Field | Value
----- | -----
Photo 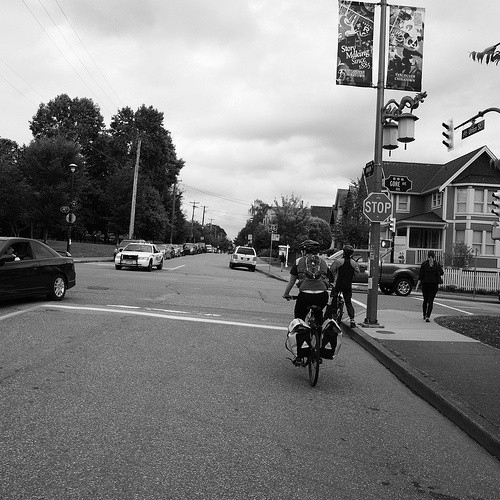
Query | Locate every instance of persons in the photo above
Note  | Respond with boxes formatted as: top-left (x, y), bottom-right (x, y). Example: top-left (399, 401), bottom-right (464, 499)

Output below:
top-left (5, 246), bottom-right (27, 260)
top-left (323, 245), bottom-right (360, 328)
top-left (416, 250), bottom-right (445, 323)
top-left (280, 252), bottom-right (285, 271)
top-left (183, 244), bottom-right (186, 250)
top-left (398, 252), bottom-right (404, 263)
top-left (284, 240), bottom-right (335, 366)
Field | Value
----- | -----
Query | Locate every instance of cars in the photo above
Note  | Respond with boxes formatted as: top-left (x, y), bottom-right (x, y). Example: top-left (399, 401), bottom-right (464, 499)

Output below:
top-left (158, 243), bottom-right (207, 260)
top-left (115, 242), bottom-right (164, 271)
top-left (0, 236), bottom-right (77, 301)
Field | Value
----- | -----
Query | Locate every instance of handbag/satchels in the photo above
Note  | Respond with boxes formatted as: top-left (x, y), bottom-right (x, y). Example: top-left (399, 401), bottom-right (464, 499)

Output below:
top-left (285, 318), bottom-right (317, 358)
top-left (437, 261), bottom-right (443, 284)
top-left (319, 318), bottom-right (343, 360)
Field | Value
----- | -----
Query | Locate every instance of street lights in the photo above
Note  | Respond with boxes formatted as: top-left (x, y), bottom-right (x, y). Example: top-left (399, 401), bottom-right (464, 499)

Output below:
top-left (67, 164), bottom-right (78, 253)
top-left (358, 90), bottom-right (428, 330)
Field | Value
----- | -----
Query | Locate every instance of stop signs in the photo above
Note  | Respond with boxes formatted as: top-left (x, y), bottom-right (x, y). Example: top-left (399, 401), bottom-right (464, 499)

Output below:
top-left (362, 192), bottom-right (393, 223)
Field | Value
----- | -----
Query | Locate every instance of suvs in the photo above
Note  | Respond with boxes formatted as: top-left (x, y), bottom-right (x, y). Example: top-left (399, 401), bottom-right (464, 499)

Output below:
top-left (229, 246), bottom-right (257, 272)
top-left (324, 249), bottom-right (421, 297)
top-left (206, 245), bottom-right (212, 252)
top-left (113, 239), bottom-right (145, 261)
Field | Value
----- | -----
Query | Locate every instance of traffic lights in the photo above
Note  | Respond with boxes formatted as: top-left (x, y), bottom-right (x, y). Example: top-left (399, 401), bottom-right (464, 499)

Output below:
top-left (490, 191), bottom-right (500, 216)
top-left (442, 120), bottom-right (455, 151)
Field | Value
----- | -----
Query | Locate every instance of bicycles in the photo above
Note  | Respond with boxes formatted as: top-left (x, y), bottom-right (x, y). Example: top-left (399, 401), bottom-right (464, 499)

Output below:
top-left (323, 282), bottom-right (345, 330)
top-left (283, 294), bottom-right (323, 388)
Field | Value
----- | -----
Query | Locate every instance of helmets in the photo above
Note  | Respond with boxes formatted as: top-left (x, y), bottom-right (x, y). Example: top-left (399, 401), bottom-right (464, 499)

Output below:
top-left (343, 245), bottom-right (354, 253)
top-left (299, 240), bottom-right (320, 254)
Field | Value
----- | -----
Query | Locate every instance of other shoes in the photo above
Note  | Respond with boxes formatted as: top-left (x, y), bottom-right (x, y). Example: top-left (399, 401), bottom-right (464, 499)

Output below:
top-left (315, 357), bottom-right (322, 364)
top-left (295, 358), bottom-right (302, 364)
top-left (350, 320), bottom-right (356, 329)
top-left (425, 319), bottom-right (430, 322)
top-left (423, 316), bottom-right (426, 320)
